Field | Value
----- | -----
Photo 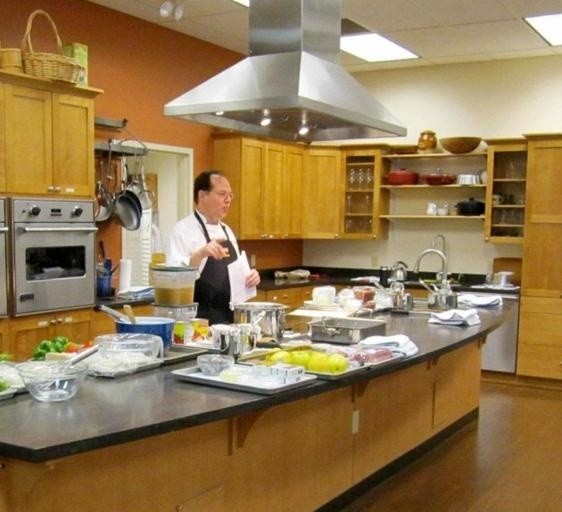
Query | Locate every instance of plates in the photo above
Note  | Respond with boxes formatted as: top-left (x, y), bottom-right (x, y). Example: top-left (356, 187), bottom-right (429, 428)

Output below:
top-left (171, 363), bottom-right (319, 396)
top-left (237, 346), bottom-right (373, 382)
top-left (54, 344), bottom-right (210, 379)
top-left (303, 301), bottom-right (341, 313)
top-left (0, 358), bottom-right (31, 403)
top-left (367, 350), bottom-right (406, 369)
top-left (175, 335), bottom-right (230, 354)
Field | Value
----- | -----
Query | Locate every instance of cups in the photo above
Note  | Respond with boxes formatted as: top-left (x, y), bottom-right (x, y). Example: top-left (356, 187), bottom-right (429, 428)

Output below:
top-left (492, 194), bottom-right (505, 207)
top-left (313, 286), bottom-right (337, 305)
top-left (347, 168), bottom-right (374, 188)
top-left (380, 267), bottom-right (389, 288)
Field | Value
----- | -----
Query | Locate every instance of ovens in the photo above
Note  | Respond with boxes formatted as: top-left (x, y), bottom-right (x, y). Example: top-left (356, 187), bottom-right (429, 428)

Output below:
top-left (0, 197), bottom-right (10, 318)
top-left (12, 197), bottom-right (96, 317)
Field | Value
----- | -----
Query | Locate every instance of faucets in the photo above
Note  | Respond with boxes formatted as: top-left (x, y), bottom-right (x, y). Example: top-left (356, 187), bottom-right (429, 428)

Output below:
top-left (432, 234), bottom-right (446, 256)
top-left (414, 250), bottom-right (448, 309)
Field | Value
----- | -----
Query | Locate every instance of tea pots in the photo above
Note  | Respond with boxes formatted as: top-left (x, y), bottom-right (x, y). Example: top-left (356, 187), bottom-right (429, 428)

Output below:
top-left (427, 202), bottom-right (438, 217)
top-left (372, 280), bottom-right (414, 313)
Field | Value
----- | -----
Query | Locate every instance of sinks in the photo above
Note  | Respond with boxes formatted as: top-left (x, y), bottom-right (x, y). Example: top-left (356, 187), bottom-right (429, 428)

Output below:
top-left (411, 301), bottom-right (437, 312)
top-left (405, 280), bottom-right (460, 286)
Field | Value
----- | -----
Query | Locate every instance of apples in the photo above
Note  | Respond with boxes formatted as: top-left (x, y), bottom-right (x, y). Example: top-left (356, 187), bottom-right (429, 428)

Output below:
top-left (326, 354), bottom-right (348, 374)
top-left (288, 350), bottom-right (310, 366)
top-left (307, 351), bottom-right (330, 373)
top-left (269, 349), bottom-right (288, 365)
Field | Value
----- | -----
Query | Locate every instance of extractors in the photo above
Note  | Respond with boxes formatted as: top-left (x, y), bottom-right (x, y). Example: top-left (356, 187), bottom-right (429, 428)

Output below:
top-left (163, 3), bottom-right (409, 145)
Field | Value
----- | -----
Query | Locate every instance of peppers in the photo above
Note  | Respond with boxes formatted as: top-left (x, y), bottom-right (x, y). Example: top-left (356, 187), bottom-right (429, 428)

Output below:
top-left (33, 335), bottom-right (69, 360)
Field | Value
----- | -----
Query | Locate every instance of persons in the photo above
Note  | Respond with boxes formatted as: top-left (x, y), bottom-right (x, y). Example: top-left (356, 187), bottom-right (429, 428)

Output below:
top-left (168, 172), bottom-right (260, 325)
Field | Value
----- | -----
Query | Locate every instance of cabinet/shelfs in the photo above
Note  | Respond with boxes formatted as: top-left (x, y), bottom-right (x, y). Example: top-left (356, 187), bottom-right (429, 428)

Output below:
top-left (334, 285), bottom-right (347, 296)
top-left (304, 149), bottom-right (388, 240)
top-left (0, 80), bottom-right (95, 198)
top-left (212, 137), bottom-right (306, 239)
top-left (9, 308), bottom-right (93, 364)
top-left (93, 305), bottom-right (154, 337)
top-left (403, 289), bottom-right (438, 301)
top-left (248, 287), bottom-right (325, 335)
top-left (379, 152), bottom-right (488, 220)
top-left (482, 138), bottom-right (528, 245)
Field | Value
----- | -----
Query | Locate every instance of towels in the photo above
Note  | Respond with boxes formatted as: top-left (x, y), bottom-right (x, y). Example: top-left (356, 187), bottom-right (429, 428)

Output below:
top-left (358, 334), bottom-right (419, 359)
top-left (457, 292), bottom-right (502, 308)
top-left (427, 308), bottom-right (481, 326)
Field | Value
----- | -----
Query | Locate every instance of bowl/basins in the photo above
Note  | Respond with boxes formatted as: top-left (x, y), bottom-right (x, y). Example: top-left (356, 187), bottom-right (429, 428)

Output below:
top-left (17, 359), bottom-right (90, 403)
top-left (438, 208), bottom-right (448, 215)
top-left (94, 333), bottom-right (162, 365)
top-left (196, 353), bottom-right (234, 376)
top-left (233, 302), bottom-right (286, 348)
top-left (454, 175), bottom-right (483, 189)
top-left (396, 146), bottom-right (417, 155)
top-left (311, 308), bottom-right (387, 346)
top-left (440, 137), bottom-right (482, 155)
top-left (115, 315), bottom-right (176, 357)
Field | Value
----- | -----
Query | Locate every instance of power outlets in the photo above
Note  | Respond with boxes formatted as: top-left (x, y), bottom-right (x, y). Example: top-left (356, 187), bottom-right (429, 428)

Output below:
top-left (250, 254), bottom-right (256, 267)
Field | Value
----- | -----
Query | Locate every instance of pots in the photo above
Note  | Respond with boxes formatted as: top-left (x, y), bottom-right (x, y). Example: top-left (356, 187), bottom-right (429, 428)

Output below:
top-left (116, 162), bottom-right (143, 234)
top-left (418, 131), bottom-right (438, 157)
top-left (418, 169), bottom-right (456, 187)
top-left (383, 167), bottom-right (419, 185)
top-left (95, 184), bottom-right (115, 223)
top-left (454, 198), bottom-right (486, 217)
